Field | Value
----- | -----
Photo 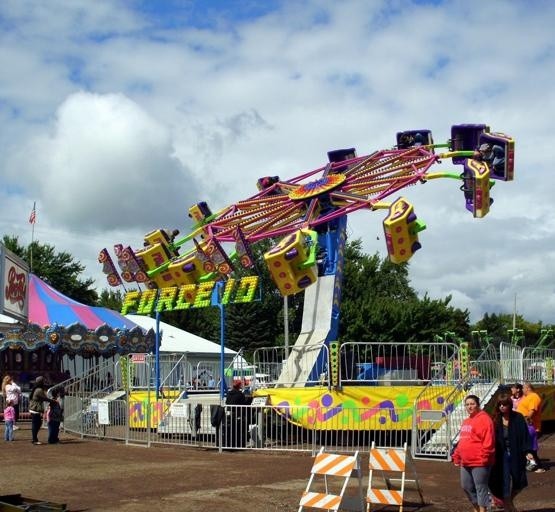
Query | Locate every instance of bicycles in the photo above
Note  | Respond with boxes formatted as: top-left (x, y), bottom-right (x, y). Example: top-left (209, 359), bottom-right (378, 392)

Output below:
top-left (77, 400), bottom-right (106, 441)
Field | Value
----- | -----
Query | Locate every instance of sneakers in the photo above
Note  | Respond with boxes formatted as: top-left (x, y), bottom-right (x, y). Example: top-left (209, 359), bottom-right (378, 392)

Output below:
top-left (32, 441), bottom-right (42, 445)
top-left (526, 464), bottom-right (545, 473)
top-left (13, 425), bottom-right (20, 430)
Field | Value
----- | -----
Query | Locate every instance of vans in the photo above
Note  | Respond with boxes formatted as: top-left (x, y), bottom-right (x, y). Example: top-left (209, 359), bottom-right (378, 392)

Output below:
top-left (431, 362), bottom-right (445, 371)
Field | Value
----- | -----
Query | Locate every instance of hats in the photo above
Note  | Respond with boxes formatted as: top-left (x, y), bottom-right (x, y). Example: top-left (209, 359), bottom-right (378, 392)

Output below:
top-left (36, 377), bottom-right (47, 384)
top-left (233, 380), bottom-right (241, 387)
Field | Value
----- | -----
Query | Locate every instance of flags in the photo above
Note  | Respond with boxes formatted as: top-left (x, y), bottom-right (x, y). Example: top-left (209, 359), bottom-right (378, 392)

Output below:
top-left (29, 203), bottom-right (36, 225)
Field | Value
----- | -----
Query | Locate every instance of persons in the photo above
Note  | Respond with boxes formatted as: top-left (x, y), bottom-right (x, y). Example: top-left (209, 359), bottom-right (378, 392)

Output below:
top-left (450, 395), bottom-right (497, 512)
top-left (508, 383), bottom-right (523, 411)
top-left (486, 392), bottom-right (536, 511)
top-left (515, 381), bottom-right (546, 474)
top-left (1, 368), bottom-right (247, 450)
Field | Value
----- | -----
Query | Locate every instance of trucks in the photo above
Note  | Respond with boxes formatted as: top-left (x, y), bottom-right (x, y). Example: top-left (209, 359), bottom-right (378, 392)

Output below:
top-left (525, 359), bottom-right (555, 381)
